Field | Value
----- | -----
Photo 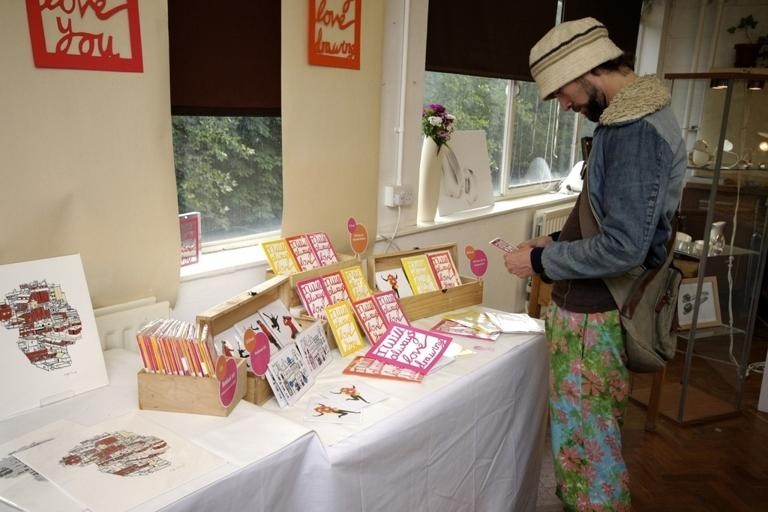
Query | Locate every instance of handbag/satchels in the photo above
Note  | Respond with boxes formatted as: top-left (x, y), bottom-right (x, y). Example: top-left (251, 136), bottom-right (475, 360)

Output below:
top-left (622, 264), bottom-right (684, 377)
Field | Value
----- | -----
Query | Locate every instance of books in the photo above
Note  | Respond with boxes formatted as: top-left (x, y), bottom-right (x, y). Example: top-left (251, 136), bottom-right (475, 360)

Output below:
top-left (136, 231), bottom-right (546, 409)
top-left (179, 212), bottom-right (201, 267)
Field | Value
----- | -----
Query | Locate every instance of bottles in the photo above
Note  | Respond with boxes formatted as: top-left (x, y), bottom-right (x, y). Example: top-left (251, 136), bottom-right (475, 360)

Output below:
top-left (706, 221), bottom-right (726, 257)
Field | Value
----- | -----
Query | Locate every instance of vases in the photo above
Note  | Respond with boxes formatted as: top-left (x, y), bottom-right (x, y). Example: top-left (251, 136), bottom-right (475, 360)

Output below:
top-left (417, 134), bottom-right (443, 223)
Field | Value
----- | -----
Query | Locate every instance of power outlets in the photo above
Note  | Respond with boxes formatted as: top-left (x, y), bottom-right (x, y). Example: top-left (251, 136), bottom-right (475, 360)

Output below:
top-left (384, 185), bottom-right (414, 208)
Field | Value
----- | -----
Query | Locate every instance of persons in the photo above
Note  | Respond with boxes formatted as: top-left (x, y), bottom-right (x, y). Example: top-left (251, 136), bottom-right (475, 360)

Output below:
top-left (502, 16), bottom-right (689, 510)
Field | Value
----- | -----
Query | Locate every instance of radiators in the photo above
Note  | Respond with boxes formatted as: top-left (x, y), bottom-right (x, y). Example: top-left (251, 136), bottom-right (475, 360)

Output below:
top-left (526, 201), bottom-right (578, 294)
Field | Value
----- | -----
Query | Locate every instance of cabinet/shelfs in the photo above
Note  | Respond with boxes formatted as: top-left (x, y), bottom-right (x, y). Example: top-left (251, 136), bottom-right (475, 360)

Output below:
top-left (621, 66), bottom-right (768, 427)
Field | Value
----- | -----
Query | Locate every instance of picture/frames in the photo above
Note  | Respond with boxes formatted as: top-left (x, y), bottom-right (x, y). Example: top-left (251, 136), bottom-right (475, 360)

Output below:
top-left (672, 276), bottom-right (722, 331)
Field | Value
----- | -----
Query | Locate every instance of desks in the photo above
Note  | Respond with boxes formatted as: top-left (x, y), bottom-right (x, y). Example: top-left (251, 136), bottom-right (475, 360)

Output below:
top-left (2, 302), bottom-right (551, 512)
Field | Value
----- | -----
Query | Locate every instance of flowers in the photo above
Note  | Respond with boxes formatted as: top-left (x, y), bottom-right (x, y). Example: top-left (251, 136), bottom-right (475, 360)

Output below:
top-left (421, 103), bottom-right (457, 159)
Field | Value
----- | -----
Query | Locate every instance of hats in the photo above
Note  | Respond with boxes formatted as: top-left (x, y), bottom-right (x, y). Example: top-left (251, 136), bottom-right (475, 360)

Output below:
top-left (529, 16), bottom-right (625, 100)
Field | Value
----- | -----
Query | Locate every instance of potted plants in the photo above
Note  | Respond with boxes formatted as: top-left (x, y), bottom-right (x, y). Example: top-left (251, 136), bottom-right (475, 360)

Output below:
top-left (725, 13), bottom-right (768, 68)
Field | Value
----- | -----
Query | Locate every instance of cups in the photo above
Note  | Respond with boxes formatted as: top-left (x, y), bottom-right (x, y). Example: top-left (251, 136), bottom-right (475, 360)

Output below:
top-left (673, 232), bottom-right (704, 258)
